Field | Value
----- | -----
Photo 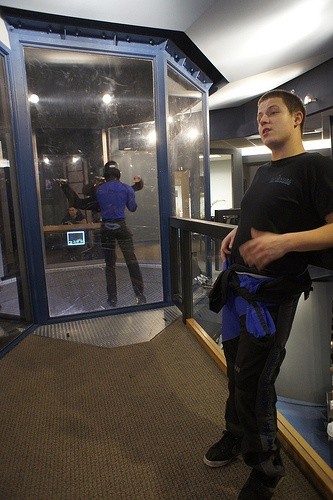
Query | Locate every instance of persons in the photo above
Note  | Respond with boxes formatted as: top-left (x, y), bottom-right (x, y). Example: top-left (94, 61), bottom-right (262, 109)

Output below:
top-left (94, 160), bottom-right (148, 306)
top-left (53, 175), bottom-right (144, 212)
top-left (62, 203), bottom-right (87, 262)
top-left (203, 89), bottom-right (333, 500)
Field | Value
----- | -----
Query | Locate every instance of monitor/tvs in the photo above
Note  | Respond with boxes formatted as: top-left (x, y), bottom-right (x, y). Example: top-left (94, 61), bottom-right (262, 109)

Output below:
top-left (67, 231), bottom-right (85, 245)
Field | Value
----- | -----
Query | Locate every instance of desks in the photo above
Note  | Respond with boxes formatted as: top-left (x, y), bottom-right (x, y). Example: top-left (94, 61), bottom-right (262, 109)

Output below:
top-left (43, 223), bottom-right (102, 249)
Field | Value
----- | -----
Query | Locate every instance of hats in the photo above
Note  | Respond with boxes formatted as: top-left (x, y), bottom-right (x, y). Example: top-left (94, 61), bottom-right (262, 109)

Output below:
top-left (104, 161), bottom-right (120, 177)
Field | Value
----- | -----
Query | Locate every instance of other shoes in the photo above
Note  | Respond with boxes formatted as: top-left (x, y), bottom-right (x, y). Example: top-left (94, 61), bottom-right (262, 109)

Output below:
top-left (136, 294), bottom-right (146, 304)
top-left (107, 297), bottom-right (117, 307)
top-left (236, 466), bottom-right (286, 500)
top-left (203, 430), bottom-right (245, 467)
